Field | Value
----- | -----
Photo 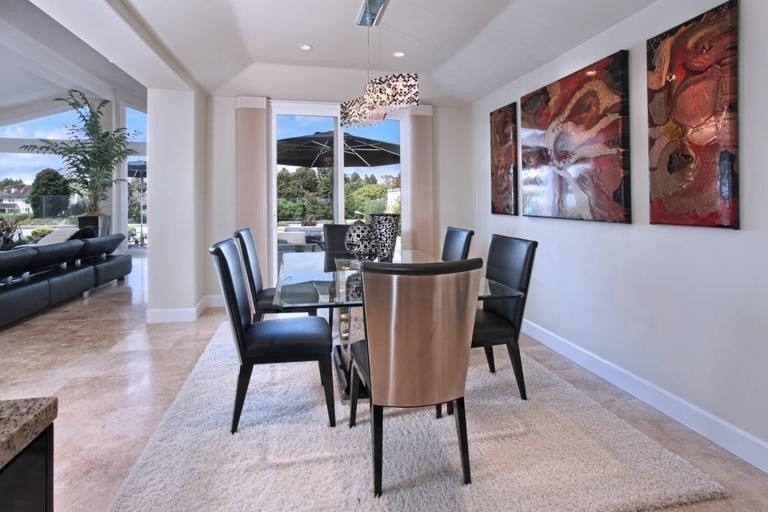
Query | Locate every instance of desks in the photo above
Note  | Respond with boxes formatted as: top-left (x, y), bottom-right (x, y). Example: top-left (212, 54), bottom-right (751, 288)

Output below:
top-left (332, 248), bottom-right (439, 402)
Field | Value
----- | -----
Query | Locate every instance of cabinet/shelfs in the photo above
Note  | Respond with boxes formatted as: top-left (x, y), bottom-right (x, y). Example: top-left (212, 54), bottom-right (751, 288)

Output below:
top-left (0, 422), bottom-right (54, 512)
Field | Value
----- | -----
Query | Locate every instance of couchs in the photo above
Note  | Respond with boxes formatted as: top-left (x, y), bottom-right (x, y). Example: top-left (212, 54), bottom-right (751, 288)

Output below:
top-left (1, 233), bottom-right (131, 328)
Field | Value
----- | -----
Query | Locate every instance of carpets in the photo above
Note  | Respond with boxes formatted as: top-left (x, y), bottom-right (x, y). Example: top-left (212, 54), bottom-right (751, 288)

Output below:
top-left (106, 320), bottom-right (732, 512)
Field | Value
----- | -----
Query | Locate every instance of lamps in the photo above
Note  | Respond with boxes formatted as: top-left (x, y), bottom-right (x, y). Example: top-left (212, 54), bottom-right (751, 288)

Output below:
top-left (340, 20), bottom-right (388, 128)
top-left (363, 0), bottom-right (420, 113)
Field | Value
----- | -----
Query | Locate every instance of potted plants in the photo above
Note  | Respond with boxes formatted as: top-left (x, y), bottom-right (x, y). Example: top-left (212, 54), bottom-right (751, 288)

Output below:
top-left (18, 90), bottom-right (143, 237)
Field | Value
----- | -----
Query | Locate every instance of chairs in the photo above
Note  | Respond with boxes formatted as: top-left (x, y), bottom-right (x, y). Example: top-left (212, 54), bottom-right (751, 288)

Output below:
top-left (233, 227), bottom-right (319, 323)
top-left (471, 234), bottom-right (541, 400)
top-left (350, 258), bottom-right (483, 498)
top-left (208, 238), bottom-right (336, 433)
top-left (442, 226), bottom-right (474, 262)
top-left (65, 225), bottom-right (99, 241)
top-left (324, 224), bottom-right (368, 331)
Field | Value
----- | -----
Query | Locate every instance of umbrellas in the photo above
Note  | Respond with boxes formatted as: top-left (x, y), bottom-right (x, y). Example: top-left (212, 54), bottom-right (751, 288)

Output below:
top-left (127, 161), bottom-right (147, 244)
top-left (277, 131), bottom-right (400, 167)
